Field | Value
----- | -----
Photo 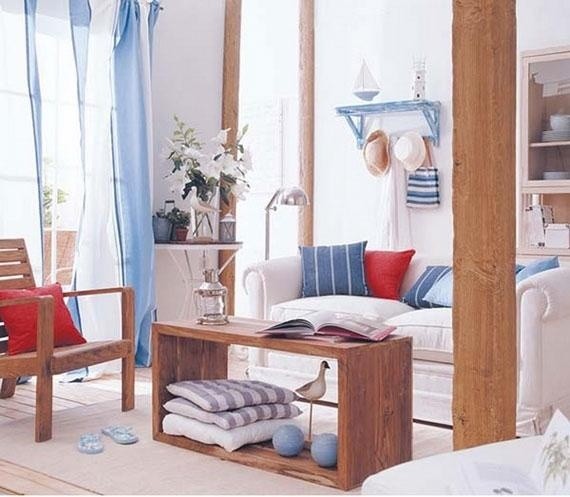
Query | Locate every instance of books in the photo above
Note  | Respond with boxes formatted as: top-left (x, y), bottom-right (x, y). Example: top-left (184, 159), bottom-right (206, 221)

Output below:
top-left (525, 202), bottom-right (555, 250)
top-left (255, 312), bottom-right (398, 341)
top-left (458, 410), bottom-right (570, 493)
top-left (284, 332), bottom-right (349, 346)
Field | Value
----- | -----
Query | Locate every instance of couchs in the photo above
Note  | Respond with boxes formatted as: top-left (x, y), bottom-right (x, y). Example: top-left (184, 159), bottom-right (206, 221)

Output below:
top-left (242, 255), bottom-right (569, 439)
top-left (362, 435), bottom-right (568, 495)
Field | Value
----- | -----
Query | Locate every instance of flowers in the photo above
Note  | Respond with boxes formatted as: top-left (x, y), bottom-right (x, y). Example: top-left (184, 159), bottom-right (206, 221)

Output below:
top-left (161, 115), bottom-right (252, 237)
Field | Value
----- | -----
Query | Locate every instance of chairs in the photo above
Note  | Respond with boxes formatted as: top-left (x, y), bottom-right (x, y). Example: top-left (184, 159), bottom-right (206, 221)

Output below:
top-left (0, 237), bottom-right (135, 443)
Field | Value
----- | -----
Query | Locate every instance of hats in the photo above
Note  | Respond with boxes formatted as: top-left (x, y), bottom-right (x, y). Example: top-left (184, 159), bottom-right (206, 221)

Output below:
top-left (393, 131), bottom-right (426, 172)
top-left (362, 129), bottom-right (390, 179)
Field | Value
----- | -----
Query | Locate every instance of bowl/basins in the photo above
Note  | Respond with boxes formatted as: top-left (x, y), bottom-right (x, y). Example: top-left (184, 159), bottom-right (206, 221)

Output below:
top-left (548, 112), bottom-right (570, 131)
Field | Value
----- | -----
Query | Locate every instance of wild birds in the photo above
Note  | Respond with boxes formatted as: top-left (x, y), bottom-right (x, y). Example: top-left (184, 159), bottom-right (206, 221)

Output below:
top-left (294, 361), bottom-right (331, 400)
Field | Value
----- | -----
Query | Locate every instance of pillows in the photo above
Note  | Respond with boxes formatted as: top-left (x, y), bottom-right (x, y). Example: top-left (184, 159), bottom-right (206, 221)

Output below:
top-left (405, 265), bottom-right (453, 308)
top-left (529, 403), bottom-right (569, 496)
top-left (420, 265), bottom-right (522, 307)
top-left (514, 256), bottom-right (558, 283)
top-left (364, 248), bottom-right (414, 301)
top-left (0, 281), bottom-right (87, 357)
top-left (297, 240), bottom-right (367, 297)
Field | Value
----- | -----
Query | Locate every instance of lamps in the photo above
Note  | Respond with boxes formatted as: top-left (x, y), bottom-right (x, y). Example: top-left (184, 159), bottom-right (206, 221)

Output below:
top-left (193, 269), bottom-right (230, 327)
top-left (263, 185), bottom-right (321, 263)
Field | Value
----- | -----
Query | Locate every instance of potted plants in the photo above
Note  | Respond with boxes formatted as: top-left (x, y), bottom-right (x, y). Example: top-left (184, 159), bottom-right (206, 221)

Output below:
top-left (165, 206), bottom-right (193, 242)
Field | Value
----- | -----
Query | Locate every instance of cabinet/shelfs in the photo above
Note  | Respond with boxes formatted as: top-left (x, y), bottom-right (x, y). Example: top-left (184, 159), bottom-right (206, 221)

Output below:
top-left (516, 45), bottom-right (569, 258)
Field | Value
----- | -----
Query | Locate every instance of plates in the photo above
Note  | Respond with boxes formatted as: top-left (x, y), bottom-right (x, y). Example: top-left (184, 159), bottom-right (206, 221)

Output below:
top-left (542, 170), bottom-right (570, 181)
top-left (539, 129), bottom-right (570, 144)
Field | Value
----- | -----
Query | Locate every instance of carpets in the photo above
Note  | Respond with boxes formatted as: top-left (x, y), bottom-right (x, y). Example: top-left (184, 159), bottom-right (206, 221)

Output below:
top-left (1, 395), bottom-right (451, 494)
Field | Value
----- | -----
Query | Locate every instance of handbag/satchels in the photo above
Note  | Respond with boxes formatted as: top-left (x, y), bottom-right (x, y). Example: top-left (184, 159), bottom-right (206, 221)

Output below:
top-left (405, 167), bottom-right (440, 210)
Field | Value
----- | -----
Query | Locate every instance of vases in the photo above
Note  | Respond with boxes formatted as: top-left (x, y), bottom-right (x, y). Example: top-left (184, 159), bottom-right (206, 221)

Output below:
top-left (191, 205), bottom-right (216, 238)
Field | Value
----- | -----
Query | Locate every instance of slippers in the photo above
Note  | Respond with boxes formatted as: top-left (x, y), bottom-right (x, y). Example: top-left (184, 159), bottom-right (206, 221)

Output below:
top-left (101, 423), bottom-right (139, 444)
top-left (78, 431), bottom-right (103, 454)
